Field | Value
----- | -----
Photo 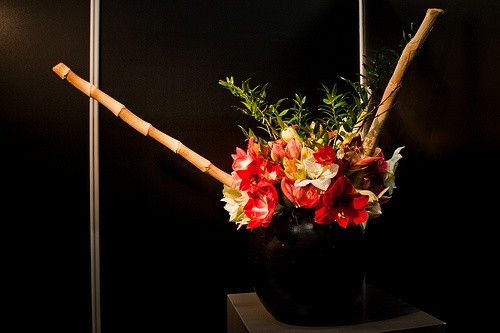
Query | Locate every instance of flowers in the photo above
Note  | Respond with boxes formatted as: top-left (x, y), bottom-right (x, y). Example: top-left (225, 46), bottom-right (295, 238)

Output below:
top-left (205, 53), bottom-right (405, 231)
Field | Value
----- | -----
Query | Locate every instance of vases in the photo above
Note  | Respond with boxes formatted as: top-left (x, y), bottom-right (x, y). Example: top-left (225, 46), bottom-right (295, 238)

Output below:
top-left (250, 210), bottom-right (406, 328)
